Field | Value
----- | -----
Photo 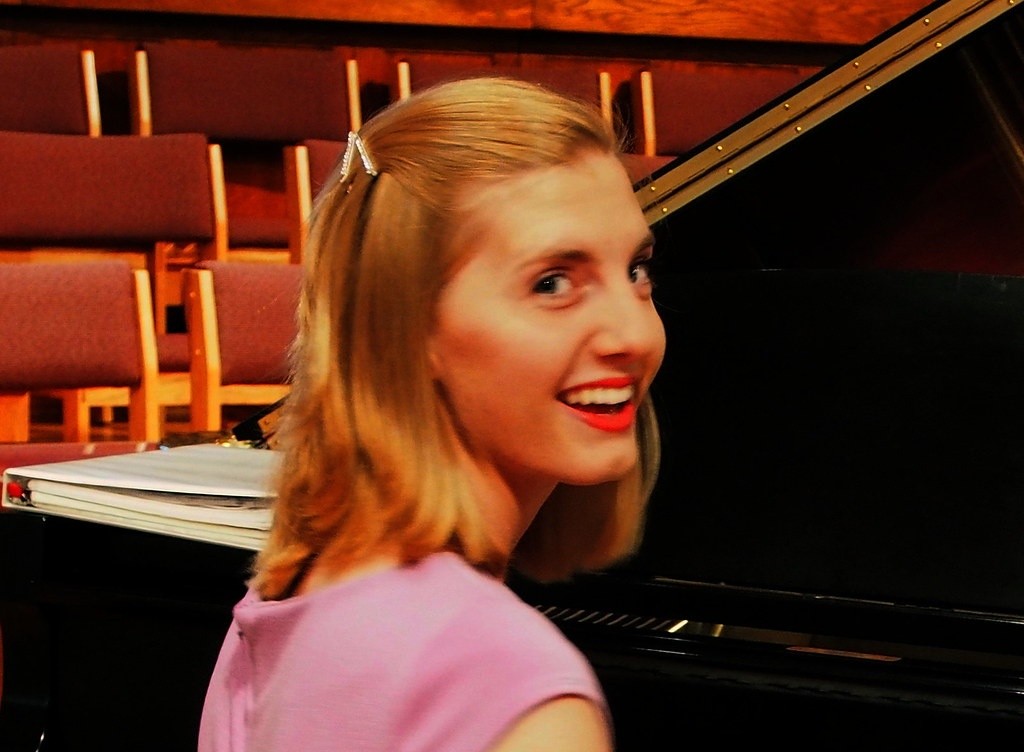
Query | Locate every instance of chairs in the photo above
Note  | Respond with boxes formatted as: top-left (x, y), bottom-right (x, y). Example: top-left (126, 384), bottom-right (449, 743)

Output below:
top-left (0, 36), bottom-right (827, 475)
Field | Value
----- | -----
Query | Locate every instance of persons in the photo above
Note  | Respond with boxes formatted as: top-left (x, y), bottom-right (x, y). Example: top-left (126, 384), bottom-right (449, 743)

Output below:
top-left (198, 75), bottom-right (665, 752)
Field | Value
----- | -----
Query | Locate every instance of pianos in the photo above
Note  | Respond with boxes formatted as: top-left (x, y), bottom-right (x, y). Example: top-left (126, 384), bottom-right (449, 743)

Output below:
top-left (0, 0), bottom-right (1023, 752)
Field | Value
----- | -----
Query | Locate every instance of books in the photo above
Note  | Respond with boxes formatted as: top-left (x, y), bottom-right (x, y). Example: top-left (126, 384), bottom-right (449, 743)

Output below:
top-left (29, 478), bottom-right (274, 551)
